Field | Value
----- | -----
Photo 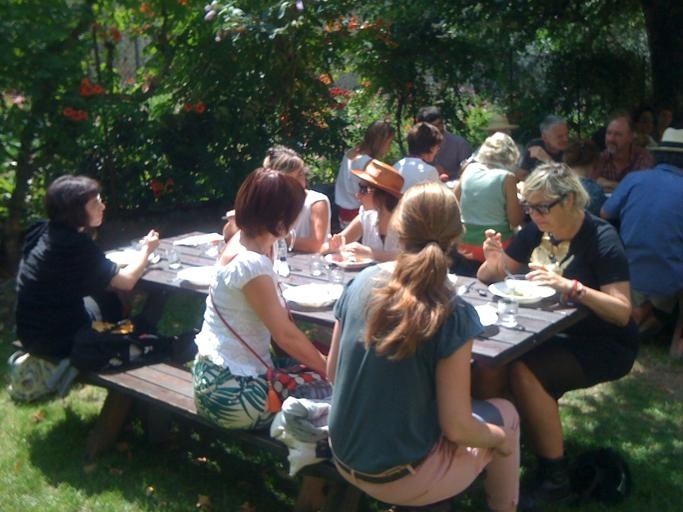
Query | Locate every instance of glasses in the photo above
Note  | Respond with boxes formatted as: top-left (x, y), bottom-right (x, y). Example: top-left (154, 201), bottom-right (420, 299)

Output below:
top-left (519, 192), bottom-right (569, 217)
top-left (357, 182), bottom-right (375, 197)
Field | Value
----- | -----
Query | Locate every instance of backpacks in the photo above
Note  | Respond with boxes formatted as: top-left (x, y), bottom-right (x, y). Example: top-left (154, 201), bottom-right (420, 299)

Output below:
top-left (207, 251), bottom-right (334, 412)
top-left (70, 311), bottom-right (169, 373)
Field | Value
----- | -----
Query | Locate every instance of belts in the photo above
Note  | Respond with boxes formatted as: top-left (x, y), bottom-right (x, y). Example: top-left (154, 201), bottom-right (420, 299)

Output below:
top-left (328, 452), bottom-right (431, 486)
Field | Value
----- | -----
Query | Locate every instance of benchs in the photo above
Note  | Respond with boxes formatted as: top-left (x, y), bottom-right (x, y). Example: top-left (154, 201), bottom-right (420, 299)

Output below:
top-left (13, 338), bottom-right (456, 512)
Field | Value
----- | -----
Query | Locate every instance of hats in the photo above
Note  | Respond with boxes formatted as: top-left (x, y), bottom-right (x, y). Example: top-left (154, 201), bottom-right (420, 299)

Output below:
top-left (349, 159), bottom-right (405, 199)
top-left (644, 125), bottom-right (683, 153)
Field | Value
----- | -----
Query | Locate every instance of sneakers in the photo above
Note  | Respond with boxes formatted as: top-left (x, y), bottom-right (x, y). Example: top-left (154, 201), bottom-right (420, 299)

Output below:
top-left (518, 479), bottom-right (579, 510)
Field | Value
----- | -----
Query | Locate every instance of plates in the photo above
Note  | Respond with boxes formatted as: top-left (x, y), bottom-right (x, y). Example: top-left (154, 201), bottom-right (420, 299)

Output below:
top-left (474, 305), bottom-right (498, 327)
top-left (282, 284), bottom-right (343, 307)
top-left (324, 253), bottom-right (373, 268)
top-left (105, 251), bottom-right (140, 267)
top-left (488, 280), bottom-right (556, 304)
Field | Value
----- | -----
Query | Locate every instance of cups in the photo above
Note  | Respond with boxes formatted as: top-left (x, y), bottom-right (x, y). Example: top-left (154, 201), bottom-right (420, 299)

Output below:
top-left (328, 266), bottom-right (345, 285)
top-left (310, 255), bottom-right (322, 276)
top-left (167, 248), bottom-right (180, 269)
top-left (496, 299), bottom-right (519, 328)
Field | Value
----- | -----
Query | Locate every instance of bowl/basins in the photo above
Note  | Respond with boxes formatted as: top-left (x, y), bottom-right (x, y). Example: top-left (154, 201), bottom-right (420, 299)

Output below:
top-left (504, 274), bottom-right (539, 294)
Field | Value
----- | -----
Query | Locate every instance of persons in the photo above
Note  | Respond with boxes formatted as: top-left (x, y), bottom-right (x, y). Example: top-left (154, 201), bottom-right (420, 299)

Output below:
top-left (325, 179), bottom-right (520, 512)
top-left (390, 121), bottom-right (444, 199)
top-left (223, 144), bottom-right (332, 253)
top-left (478, 162), bottom-right (640, 512)
top-left (649, 109), bottom-right (673, 144)
top-left (591, 114), bottom-right (655, 194)
top-left (660, 106), bottom-right (683, 143)
top-left (191, 168), bottom-right (334, 511)
top-left (450, 132), bottom-right (530, 261)
top-left (335, 119), bottom-right (397, 231)
top-left (628, 112), bottom-right (658, 149)
top-left (558, 140), bottom-right (607, 218)
top-left (16, 174), bottom-right (160, 362)
top-left (413, 105), bottom-right (472, 179)
top-left (326, 158), bottom-right (405, 262)
top-left (600, 127), bottom-right (683, 332)
top-left (518, 114), bottom-right (570, 180)
